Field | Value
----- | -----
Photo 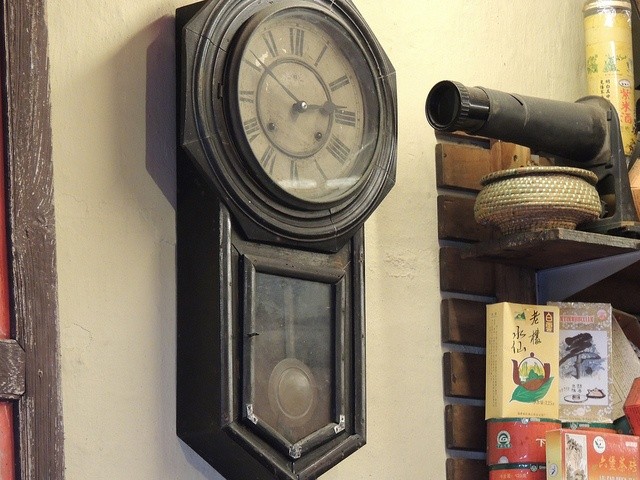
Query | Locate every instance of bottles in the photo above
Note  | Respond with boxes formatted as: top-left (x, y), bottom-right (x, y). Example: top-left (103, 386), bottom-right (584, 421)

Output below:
top-left (584, 1), bottom-right (637, 159)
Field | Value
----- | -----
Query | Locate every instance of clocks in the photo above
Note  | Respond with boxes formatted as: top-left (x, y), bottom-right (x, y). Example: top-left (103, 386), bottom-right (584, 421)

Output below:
top-left (173, 0), bottom-right (399, 480)
